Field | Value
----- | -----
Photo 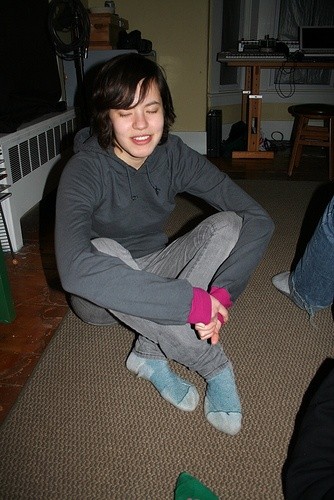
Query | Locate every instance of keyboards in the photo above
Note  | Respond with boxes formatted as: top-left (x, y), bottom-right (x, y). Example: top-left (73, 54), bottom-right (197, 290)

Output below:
top-left (294, 56), bottom-right (334, 62)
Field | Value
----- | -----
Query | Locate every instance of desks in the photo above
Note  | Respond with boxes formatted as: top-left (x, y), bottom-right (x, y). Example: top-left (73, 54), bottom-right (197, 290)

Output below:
top-left (227, 61), bottom-right (334, 157)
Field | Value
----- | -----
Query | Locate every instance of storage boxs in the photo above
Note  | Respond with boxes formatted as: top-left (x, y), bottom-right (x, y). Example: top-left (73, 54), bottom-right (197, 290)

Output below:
top-left (88, 13), bottom-right (120, 45)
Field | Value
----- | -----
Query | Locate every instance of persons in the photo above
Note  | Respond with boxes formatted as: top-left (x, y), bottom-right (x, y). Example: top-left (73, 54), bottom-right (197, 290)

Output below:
top-left (271, 192), bottom-right (333, 314)
top-left (52, 51), bottom-right (276, 435)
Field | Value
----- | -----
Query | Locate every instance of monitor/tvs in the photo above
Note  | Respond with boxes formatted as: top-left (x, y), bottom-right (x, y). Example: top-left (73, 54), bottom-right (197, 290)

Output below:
top-left (300, 25), bottom-right (334, 51)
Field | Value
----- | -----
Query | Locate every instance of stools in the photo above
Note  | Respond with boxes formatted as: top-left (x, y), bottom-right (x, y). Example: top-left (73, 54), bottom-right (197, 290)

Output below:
top-left (288, 104), bottom-right (334, 180)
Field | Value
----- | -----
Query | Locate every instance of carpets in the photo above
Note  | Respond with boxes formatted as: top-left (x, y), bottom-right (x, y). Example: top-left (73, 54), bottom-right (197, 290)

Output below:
top-left (0, 179), bottom-right (334, 500)
